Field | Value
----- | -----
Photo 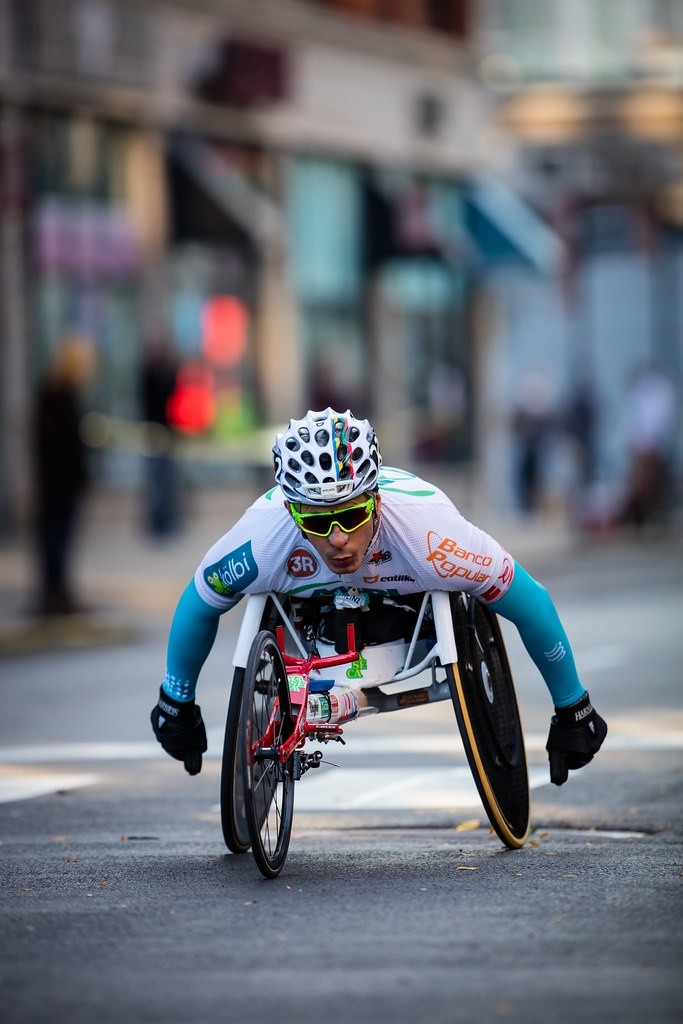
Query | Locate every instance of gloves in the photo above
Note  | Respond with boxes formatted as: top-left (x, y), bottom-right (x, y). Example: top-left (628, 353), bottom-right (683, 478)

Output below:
top-left (546, 691), bottom-right (607, 786)
top-left (150, 684), bottom-right (209, 777)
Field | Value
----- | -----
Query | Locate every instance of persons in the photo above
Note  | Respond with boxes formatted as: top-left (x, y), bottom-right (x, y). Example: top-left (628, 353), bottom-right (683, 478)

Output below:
top-left (149, 406), bottom-right (609, 787)
top-left (0, 266), bottom-right (683, 619)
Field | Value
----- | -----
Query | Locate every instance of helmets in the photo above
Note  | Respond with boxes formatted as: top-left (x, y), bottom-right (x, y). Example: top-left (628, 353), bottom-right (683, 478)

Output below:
top-left (271, 406), bottom-right (383, 506)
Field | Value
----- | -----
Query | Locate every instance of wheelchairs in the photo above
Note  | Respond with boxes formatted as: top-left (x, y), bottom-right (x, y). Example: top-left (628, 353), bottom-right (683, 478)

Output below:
top-left (220, 590), bottom-right (530, 879)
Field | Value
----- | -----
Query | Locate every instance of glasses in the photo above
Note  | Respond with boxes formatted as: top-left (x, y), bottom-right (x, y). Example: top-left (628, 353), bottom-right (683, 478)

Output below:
top-left (289, 499), bottom-right (375, 537)
